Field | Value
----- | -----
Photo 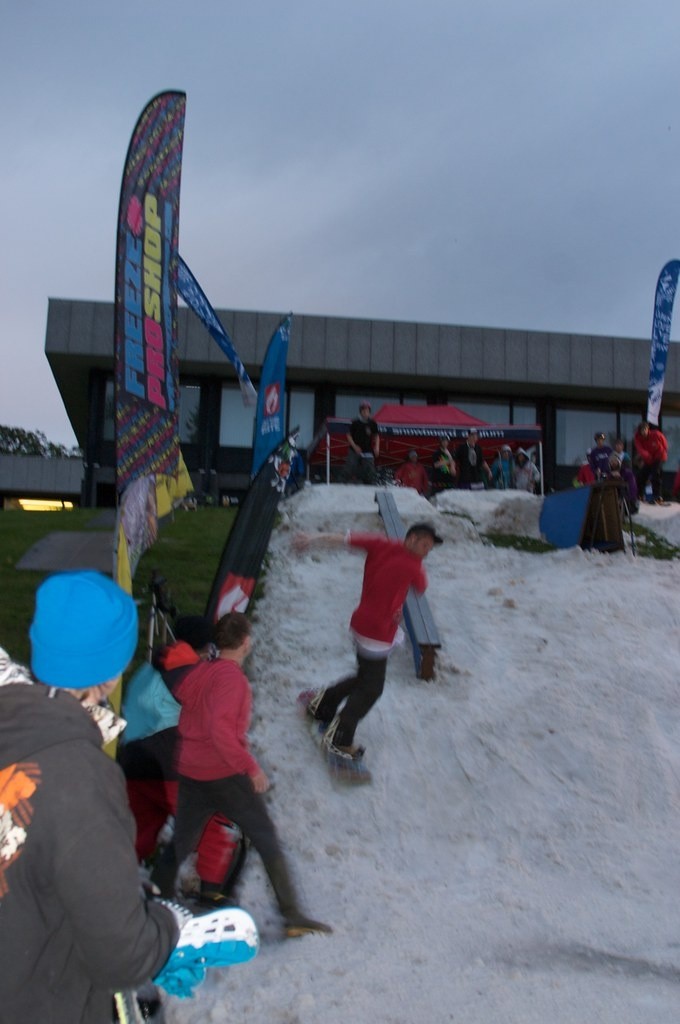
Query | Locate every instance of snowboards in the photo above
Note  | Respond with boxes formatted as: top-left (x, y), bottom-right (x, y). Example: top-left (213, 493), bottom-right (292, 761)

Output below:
top-left (300, 690), bottom-right (372, 796)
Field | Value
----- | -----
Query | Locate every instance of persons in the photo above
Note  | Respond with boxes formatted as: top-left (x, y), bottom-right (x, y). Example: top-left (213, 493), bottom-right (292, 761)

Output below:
top-left (146, 612), bottom-right (333, 934)
top-left (633, 422), bottom-right (668, 503)
top-left (426, 436), bottom-right (456, 494)
top-left (491, 443), bottom-right (514, 489)
top-left (454, 426), bottom-right (492, 489)
top-left (511, 447), bottom-right (540, 494)
top-left (575, 430), bottom-right (639, 514)
top-left (396, 449), bottom-right (428, 494)
top-left (0, 567), bottom-right (259, 1024)
top-left (346, 402), bottom-right (381, 485)
top-left (295, 521), bottom-right (443, 763)
top-left (122, 640), bottom-right (202, 855)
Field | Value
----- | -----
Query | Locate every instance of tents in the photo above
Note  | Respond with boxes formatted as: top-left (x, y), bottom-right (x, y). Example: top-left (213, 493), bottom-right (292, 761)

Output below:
top-left (308, 404), bottom-right (545, 498)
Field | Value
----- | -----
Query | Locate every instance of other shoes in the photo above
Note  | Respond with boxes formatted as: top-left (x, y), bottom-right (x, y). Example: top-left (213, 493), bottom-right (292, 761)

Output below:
top-left (197, 890), bottom-right (237, 911)
top-left (639, 494), bottom-right (645, 502)
top-left (286, 918), bottom-right (332, 937)
top-left (654, 496), bottom-right (663, 506)
top-left (329, 743), bottom-right (361, 761)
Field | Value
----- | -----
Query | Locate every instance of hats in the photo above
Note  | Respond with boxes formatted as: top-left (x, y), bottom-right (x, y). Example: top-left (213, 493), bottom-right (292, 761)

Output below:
top-left (409, 524), bottom-right (444, 545)
top-left (468, 428), bottom-right (477, 434)
top-left (163, 641), bottom-right (200, 670)
top-left (29, 569), bottom-right (138, 687)
top-left (594, 432), bottom-right (605, 439)
